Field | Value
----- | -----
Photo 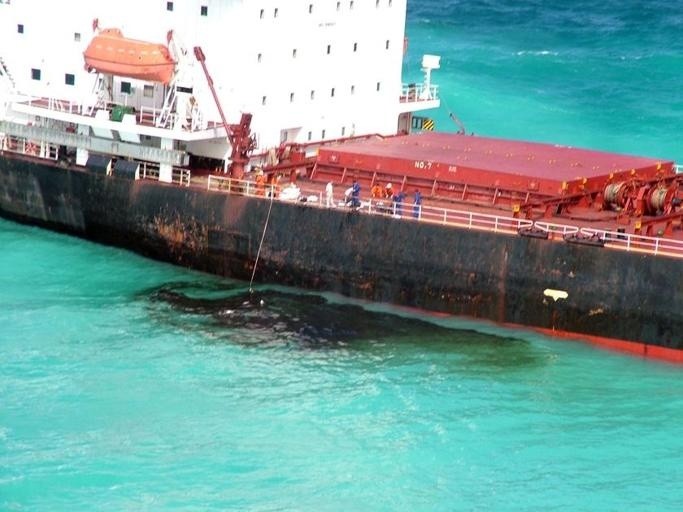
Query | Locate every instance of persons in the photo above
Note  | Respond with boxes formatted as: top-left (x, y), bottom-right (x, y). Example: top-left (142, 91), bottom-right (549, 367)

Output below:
top-left (324, 177), bottom-right (424, 221)
top-left (253, 167), bottom-right (284, 198)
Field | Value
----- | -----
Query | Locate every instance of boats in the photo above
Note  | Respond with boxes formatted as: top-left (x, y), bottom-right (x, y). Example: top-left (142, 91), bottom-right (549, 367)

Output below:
top-left (0, 0), bottom-right (683, 364)
